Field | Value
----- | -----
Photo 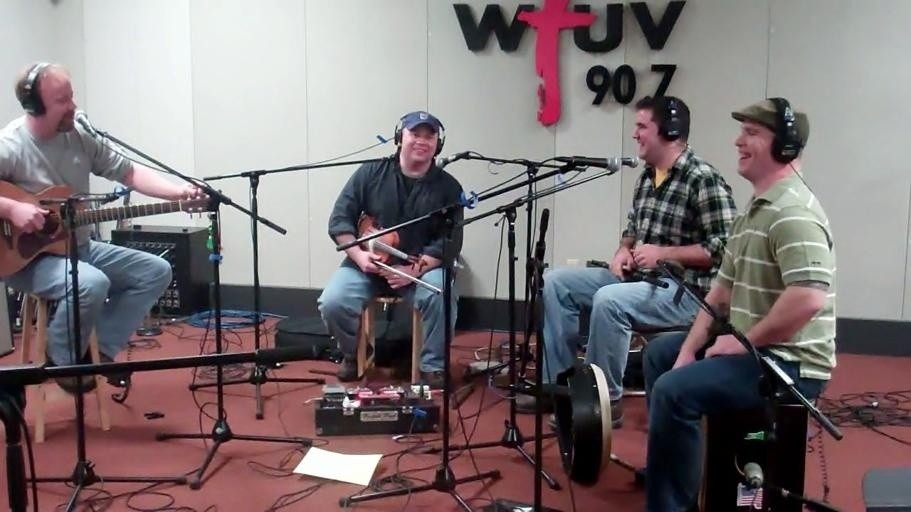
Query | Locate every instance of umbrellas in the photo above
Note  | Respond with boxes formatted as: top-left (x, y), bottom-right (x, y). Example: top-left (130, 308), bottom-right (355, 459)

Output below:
top-left (514, 381), bottom-right (570, 413)
top-left (546, 396), bottom-right (624, 433)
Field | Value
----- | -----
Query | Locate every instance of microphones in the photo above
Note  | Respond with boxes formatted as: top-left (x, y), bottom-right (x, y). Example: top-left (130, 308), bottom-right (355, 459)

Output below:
top-left (357, 294), bottom-right (421, 386)
top-left (18, 292), bottom-right (111, 442)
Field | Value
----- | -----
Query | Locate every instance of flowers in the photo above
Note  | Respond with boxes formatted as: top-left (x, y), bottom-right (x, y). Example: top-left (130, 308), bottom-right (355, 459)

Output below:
top-left (553, 155), bottom-right (625, 171)
top-left (73, 110), bottom-right (97, 138)
top-left (620, 157), bottom-right (640, 169)
top-left (436, 150), bottom-right (470, 169)
top-left (745, 461), bottom-right (764, 489)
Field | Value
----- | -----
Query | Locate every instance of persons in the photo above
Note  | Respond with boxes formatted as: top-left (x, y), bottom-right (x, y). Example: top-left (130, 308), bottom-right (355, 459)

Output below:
top-left (633, 97), bottom-right (837, 511)
top-left (515, 96), bottom-right (738, 429)
top-left (0, 61), bottom-right (210, 394)
top-left (317, 111), bottom-right (464, 391)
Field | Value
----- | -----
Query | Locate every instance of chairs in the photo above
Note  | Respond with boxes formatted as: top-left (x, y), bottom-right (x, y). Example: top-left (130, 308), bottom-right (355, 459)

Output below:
top-left (42, 349), bottom-right (96, 393)
top-left (335, 344), bottom-right (374, 381)
top-left (419, 369), bottom-right (451, 387)
top-left (83, 346), bottom-right (131, 387)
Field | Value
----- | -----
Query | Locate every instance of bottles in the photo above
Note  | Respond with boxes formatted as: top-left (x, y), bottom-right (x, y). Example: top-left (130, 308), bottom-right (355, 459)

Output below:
top-left (399, 111), bottom-right (440, 136)
top-left (729, 96), bottom-right (810, 148)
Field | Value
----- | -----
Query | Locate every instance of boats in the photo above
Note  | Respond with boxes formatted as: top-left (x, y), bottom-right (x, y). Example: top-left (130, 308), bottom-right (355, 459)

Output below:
top-left (696, 397), bottom-right (811, 512)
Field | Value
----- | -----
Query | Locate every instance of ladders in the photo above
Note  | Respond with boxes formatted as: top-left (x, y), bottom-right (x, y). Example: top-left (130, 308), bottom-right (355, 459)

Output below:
top-left (357, 216), bottom-right (427, 271)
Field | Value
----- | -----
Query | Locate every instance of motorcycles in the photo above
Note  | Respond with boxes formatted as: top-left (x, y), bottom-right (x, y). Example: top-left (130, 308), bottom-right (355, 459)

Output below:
top-left (461, 155), bottom-right (586, 383)
top-left (24, 192), bottom-right (189, 512)
top-left (420, 172), bottom-right (615, 491)
top-left (188, 157), bottom-right (388, 420)
top-left (94, 128), bottom-right (317, 486)
top-left (341, 164), bottom-right (576, 512)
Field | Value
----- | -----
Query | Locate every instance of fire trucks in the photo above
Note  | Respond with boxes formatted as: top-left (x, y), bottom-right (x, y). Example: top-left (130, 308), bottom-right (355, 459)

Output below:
top-left (588, 260), bottom-right (671, 291)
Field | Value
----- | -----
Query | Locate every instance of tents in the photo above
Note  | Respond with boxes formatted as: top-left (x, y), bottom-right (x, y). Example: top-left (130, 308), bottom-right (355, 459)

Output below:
top-left (768, 97), bottom-right (801, 164)
top-left (394, 111), bottom-right (447, 156)
top-left (663, 94), bottom-right (682, 141)
top-left (19, 63), bottom-right (49, 117)
